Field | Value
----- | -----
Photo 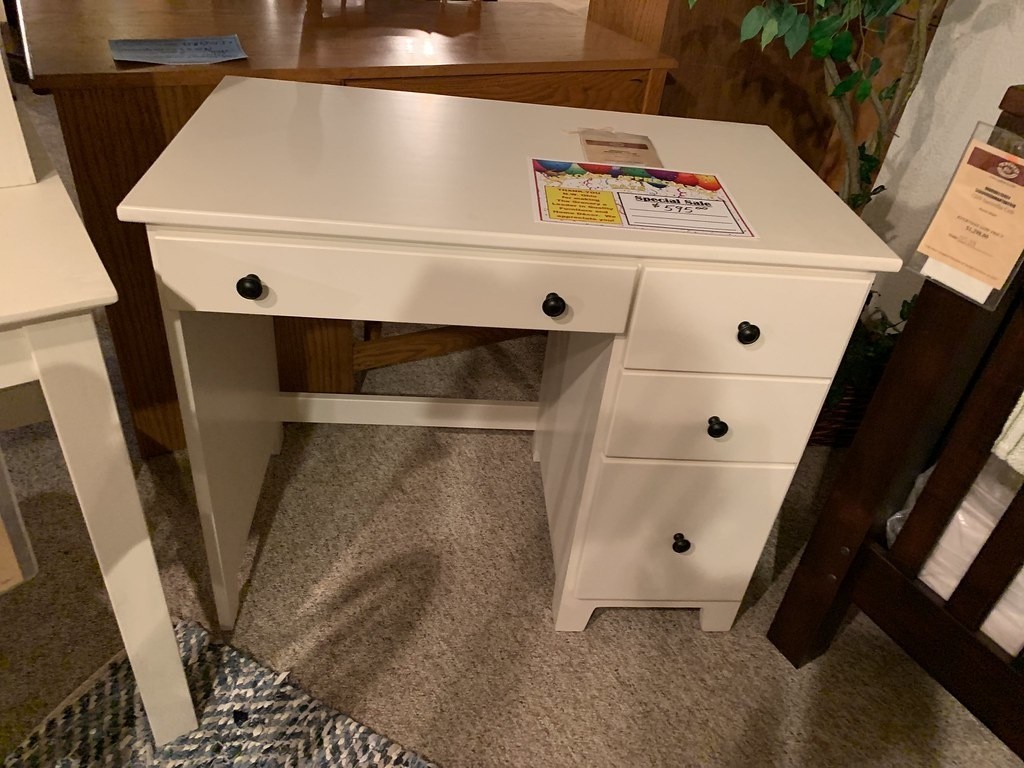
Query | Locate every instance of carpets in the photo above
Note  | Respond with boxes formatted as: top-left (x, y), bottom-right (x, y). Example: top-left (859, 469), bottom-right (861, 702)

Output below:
top-left (2, 616), bottom-right (437, 768)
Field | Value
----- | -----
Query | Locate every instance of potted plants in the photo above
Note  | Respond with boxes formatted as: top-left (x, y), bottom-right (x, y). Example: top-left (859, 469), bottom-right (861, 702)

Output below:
top-left (686, 0), bottom-right (944, 447)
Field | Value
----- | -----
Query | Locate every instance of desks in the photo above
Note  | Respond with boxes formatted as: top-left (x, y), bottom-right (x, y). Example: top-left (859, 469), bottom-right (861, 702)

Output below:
top-left (15, 1), bottom-right (680, 460)
top-left (116, 74), bottom-right (903, 634)
top-left (1, 29), bottom-right (202, 750)
top-left (762, 83), bottom-right (1024, 753)
